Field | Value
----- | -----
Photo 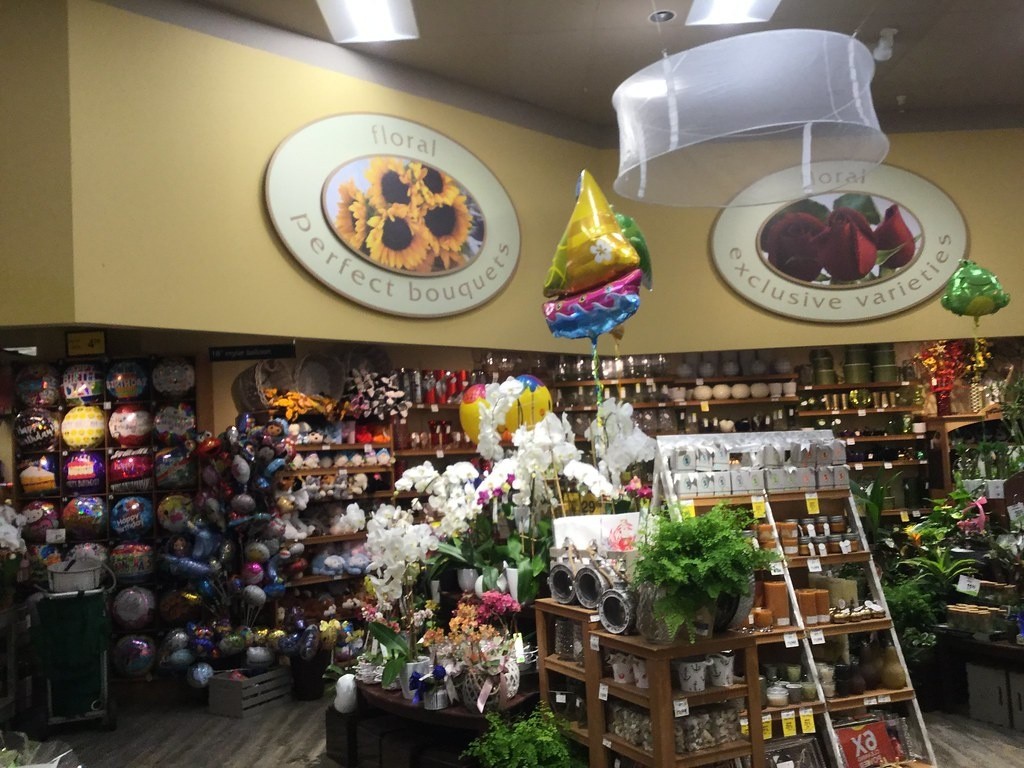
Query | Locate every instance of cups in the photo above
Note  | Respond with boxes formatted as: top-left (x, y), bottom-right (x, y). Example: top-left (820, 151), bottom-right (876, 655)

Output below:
top-left (604, 643), bottom-right (866, 763)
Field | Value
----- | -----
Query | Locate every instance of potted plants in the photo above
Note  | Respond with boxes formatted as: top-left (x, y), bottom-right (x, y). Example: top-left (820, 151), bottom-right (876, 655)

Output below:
top-left (844, 376), bottom-right (1024, 710)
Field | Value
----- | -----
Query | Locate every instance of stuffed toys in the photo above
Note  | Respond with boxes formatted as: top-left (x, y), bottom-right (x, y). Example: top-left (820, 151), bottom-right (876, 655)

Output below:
top-left (287, 416), bottom-right (393, 580)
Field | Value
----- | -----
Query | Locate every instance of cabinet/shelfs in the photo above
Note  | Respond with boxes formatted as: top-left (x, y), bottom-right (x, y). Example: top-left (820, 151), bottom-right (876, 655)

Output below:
top-left (1, 339), bottom-right (1023, 768)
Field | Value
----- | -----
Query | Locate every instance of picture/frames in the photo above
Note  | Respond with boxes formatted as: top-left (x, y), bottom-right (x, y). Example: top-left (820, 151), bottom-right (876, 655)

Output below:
top-left (260, 109), bottom-right (522, 320)
top-left (706, 158), bottom-right (970, 325)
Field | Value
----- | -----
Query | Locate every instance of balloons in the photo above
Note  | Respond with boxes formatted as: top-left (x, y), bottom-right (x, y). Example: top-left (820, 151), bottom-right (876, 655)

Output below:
top-left (17, 356), bottom-right (337, 691)
top-left (459, 374), bottom-right (551, 446)
top-left (544, 167), bottom-right (654, 345)
top-left (942, 255), bottom-right (1011, 318)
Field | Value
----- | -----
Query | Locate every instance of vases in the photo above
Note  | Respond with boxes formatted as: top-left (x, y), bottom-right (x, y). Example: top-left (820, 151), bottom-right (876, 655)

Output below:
top-left (910, 339), bottom-right (994, 420)
top-left (316, 377), bottom-right (655, 716)
top-left (637, 504), bottom-right (787, 644)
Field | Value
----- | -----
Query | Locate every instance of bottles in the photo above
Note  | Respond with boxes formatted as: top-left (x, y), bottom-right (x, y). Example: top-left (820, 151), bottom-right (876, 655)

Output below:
top-left (720, 493), bottom-right (888, 636)
top-left (546, 558), bottom-right (635, 728)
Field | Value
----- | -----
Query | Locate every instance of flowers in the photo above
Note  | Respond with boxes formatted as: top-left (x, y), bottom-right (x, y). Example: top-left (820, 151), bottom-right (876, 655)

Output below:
top-left (257, 368), bottom-right (412, 426)
